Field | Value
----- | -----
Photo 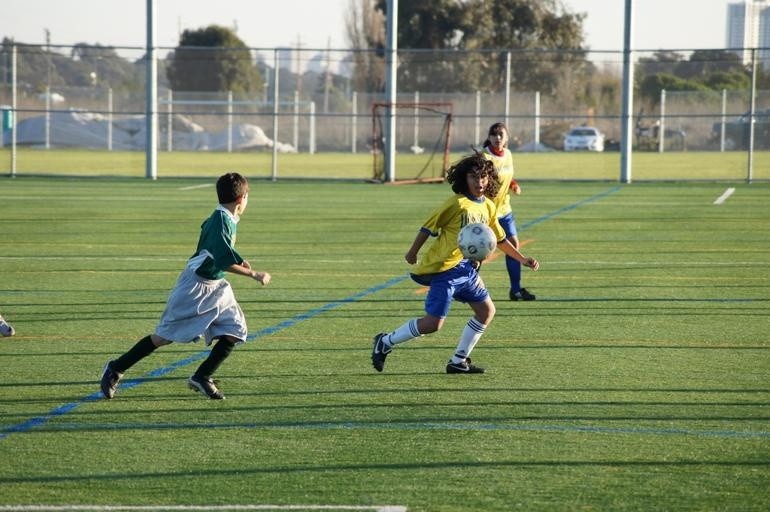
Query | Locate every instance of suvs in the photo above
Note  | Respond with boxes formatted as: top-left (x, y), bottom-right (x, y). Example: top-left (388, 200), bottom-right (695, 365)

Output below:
top-left (711, 109), bottom-right (770, 151)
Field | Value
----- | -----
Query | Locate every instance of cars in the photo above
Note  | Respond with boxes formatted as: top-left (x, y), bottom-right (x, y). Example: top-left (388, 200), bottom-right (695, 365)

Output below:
top-left (0, 78), bottom-right (66, 110)
top-left (560, 126), bottom-right (605, 152)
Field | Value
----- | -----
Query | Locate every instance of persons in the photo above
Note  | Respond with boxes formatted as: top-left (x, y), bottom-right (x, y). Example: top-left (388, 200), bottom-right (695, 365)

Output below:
top-left (370, 156), bottom-right (541, 376)
top-left (476, 121), bottom-right (538, 301)
top-left (1, 315), bottom-right (15, 337)
top-left (98, 172), bottom-right (272, 401)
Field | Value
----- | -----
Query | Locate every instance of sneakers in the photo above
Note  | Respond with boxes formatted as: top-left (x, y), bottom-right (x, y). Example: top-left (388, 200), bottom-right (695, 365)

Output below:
top-left (446, 360), bottom-right (484, 373)
top-left (371, 333), bottom-right (391, 372)
top-left (187, 373), bottom-right (224, 399)
top-left (101, 361), bottom-right (123, 398)
top-left (510, 287), bottom-right (535, 301)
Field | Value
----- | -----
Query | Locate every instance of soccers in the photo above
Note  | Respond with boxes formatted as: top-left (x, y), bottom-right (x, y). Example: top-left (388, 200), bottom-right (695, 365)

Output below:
top-left (457, 223), bottom-right (497, 260)
